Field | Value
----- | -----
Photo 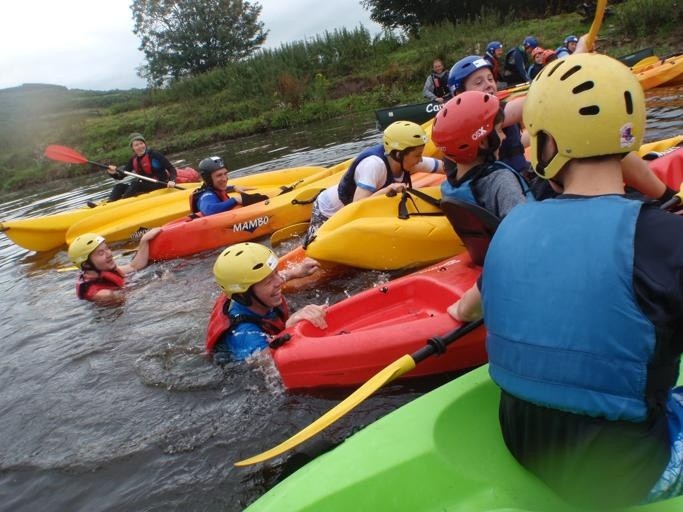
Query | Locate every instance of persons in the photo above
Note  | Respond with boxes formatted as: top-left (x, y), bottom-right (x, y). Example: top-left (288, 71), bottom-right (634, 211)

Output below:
top-left (432, 91), bottom-right (557, 218)
top-left (424, 57), bottom-right (452, 105)
top-left (187, 154), bottom-right (260, 216)
top-left (86, 133), bottom-right (175, 209)
top-left (303, 119), bottom-right (447, 245)
top-left (205, 241), bottom-right (328, 367)
top-left (447, 50), bottom-right (682, 506)
top-left (480, 35), bottom-right (579, 89)
top-left (65, 226), bottom-right (176, 305)
top-left (447, 54), bottom-right (526, 181)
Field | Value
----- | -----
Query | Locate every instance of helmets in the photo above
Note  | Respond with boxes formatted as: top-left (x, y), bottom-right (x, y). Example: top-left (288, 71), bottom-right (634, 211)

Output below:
top-left (531, 47), bottom-right (544, 59)
top-left (542, 48), bottom-right (556, 64)
top-left (447, 54), bottom-right (493, 97)
top-left (211, 241), bottom-right (280, 301)
top-left (522, 36), bottom-right (538, 49)
top-left (523, 52), bottom-right (646, 183)
top-left (382, 120), bottom-right (427, 159)
top-left (198, 156), bottom-right (230, 186)
top-left (485, 42), bottom-right (503, 54)
top-left (429, 91), bottom-right (503, 167)
top-left (66, 231), bottom-right (106, 272)
top-left (127, 132), bottom-right (146, 147)
top-left (564, 35), bottom-right (578, 48)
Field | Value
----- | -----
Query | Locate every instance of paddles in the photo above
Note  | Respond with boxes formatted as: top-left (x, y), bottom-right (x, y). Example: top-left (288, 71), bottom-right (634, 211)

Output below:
top-left (495, 83), bottom-right (531, 100)
top-left (226, 316), bottom-right (486, 469)
top-left (46, 144), bottom-right (184, 191)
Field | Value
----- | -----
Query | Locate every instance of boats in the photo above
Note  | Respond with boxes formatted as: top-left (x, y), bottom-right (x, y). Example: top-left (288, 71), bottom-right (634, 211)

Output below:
top-left (373, 46), bottom-right (655, 127)
top-left (305, 133), bottom-right (682, 271)
top-left (64, 164), bottom-right (325, 245)
top-left (234, 355), bottom-right (682, 512)
top-left (269, 146), bottom-right (683, 387)
top-left (137, 117), bottom-right (446, 261)
top-left (0, 179), bottom-right (202, 252)
top-left (496, 51), bottom-right (682, 108)
